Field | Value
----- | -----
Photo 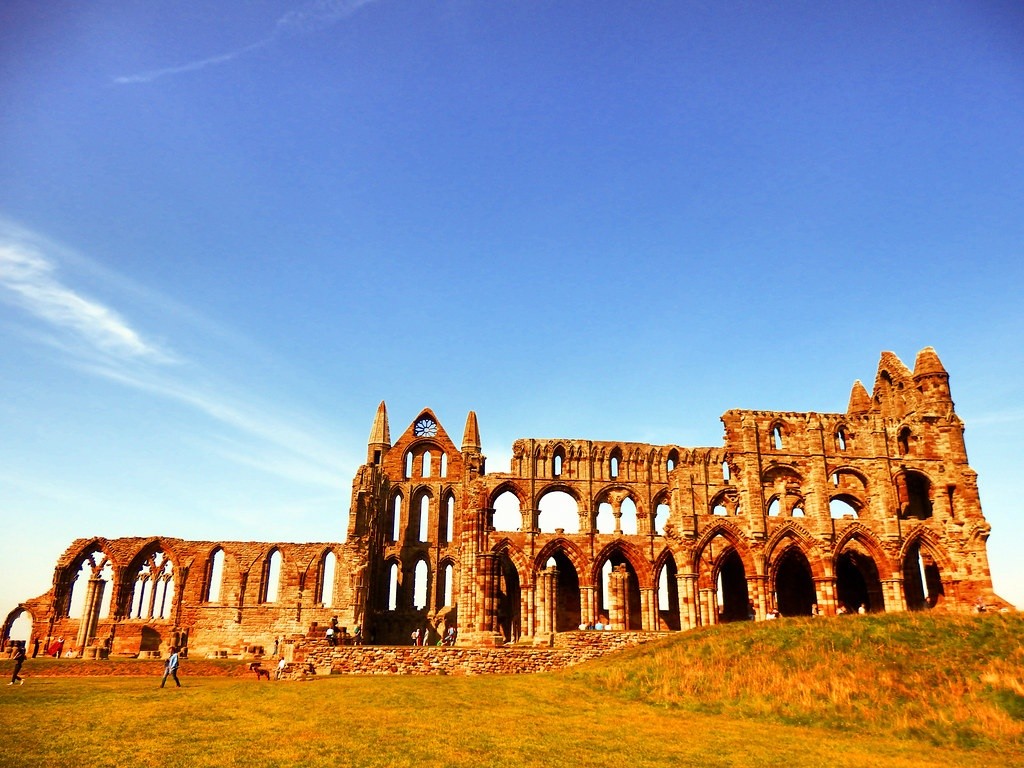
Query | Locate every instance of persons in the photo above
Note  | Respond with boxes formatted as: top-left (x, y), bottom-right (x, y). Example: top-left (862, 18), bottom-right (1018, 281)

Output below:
top-left (836, 606), bottom-right (843, 615)
top-left (3, 636), bottom-right (10, 658)
top-left (157, 646), bottom-right (181, 689)
top-left (330, 615), bottom-right (338, 629)
top-left (812, 603), bottom-right (818, 618)
top-left (411, 624), bottom-right (456, 647)
top-left (325, 626), bottom-right (338, 646)
top-left (66, 648), bottom-right (73, 657)
top-left (766, 609), bottom-right (775, 620)
top-left (858, 603), bottom-right (865, 614)
top-left (31, 636), bottom-right (42, 659)
top-left (56, 636), bottom-right (65, 659)
top-left (353, 627), bottom-right (362, 646)
top-left (277, 657), bottom-right (286, 679)
top-left (6, 648), bottom-right (25, 685)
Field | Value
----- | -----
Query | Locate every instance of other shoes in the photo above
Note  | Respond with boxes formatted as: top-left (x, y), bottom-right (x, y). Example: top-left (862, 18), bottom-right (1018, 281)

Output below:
top-left (160, 685), bottom-right (163, 688)
top-left (6, 681), bottom-right (14, 685)
top-left (176, 685), bottom-right (181, 687)
top-left (20, 679), bottom-right (25, 686)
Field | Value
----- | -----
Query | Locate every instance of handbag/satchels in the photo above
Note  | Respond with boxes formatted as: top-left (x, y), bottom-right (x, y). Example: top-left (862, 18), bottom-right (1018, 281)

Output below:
top-left (165, 660), bottom-right (169, 666)
